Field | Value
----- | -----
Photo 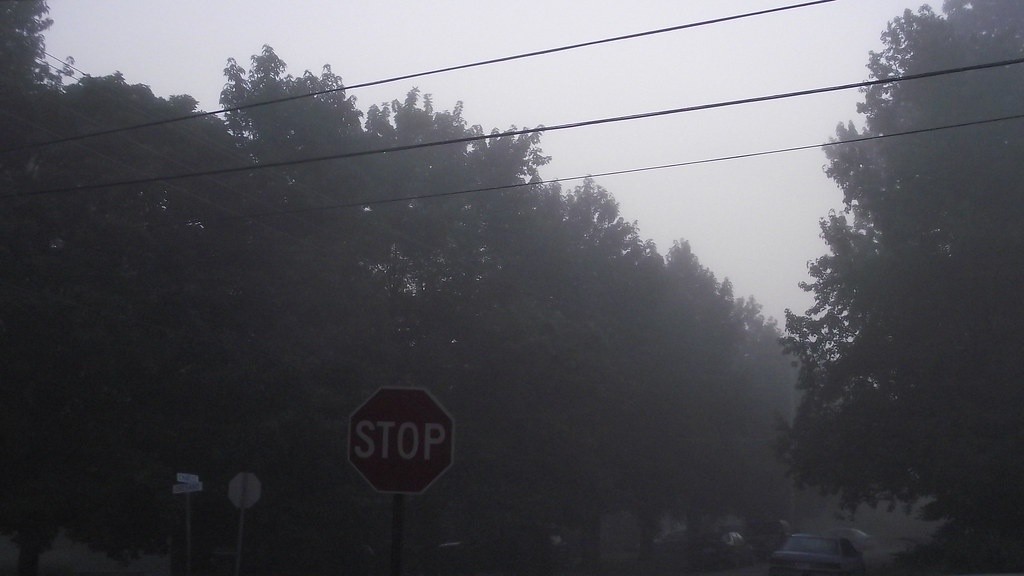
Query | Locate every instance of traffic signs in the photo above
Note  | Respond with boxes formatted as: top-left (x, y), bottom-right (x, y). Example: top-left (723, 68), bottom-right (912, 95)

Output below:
top-left (172, 473), bottom-right (203, 495)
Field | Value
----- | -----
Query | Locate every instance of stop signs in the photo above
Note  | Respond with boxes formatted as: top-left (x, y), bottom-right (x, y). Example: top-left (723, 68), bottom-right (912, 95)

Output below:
top-left (347, 386), bottom-right (456, 497)
